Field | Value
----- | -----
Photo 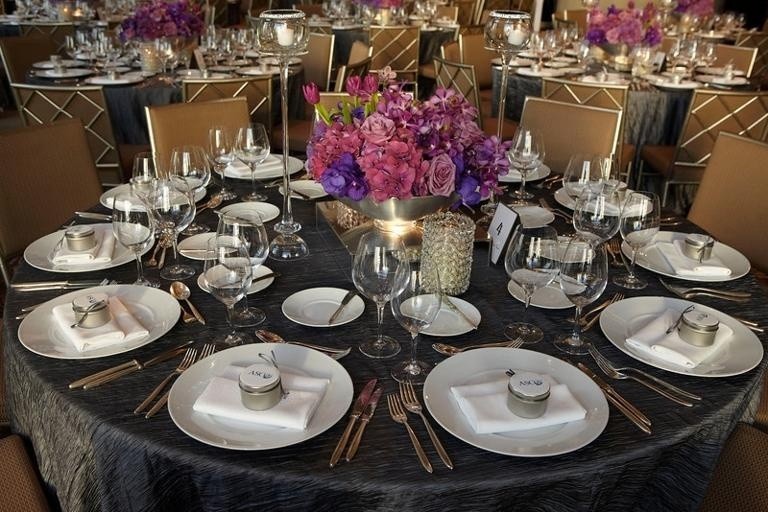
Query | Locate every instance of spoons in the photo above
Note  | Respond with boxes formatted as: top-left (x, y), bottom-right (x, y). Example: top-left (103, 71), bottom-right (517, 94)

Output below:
top-left (254, 327), bottom-right (350, 354)
top-left (169, 278), bottom-right (208, 324)
top-left (606, 243), bottom-right (624, 268)
top-left (566, 301), bottom-right (609, 326)
top-left (432, 340), bottom-right (527, 357)
top-left (143, 236), bottom-right (162, 268)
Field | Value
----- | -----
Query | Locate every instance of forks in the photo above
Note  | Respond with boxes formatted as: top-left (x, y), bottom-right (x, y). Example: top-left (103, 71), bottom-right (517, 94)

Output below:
top-left (579, 289), bottom-right (625, 334)
top-left (386, 392), bottom-right (436, 474)
top-left (608, 236), bottom-right (633, 275)
top-left (397, 379), bottom-right (455, 469)
top-left (509, 336), bottom-right (527, 349)
top-left (178, 305), bottom-right (198, 329)
top-left (584, 344), bottom-right (703, 407)
top-left (529, 174), bottom-right (564, 193)
top-left (659, 277), bottom-right (757, 304)
top-left (144, 342), bottom-right (217, 421)
top-left (132, 347), bottom-right (199, 417)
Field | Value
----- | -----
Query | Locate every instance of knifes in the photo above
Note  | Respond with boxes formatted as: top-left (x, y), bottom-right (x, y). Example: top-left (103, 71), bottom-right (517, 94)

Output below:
top-left (328, 287), bottom-right (358, 326)
top-left (276, 177), bottom-right (317, 200)
top-left (8, 278), bottom-right (129, 290)
top-left (329, 377), bottom-right (378, 468)
top-left (441, 292), bottom-right (478, 331)
top-left (576, 360), bottom-right (653, 427)
top-left (67, 339), bottom-right (197, 390)
top-left (345, 383), bottom-right (386, 463)
top-left (250, 271), bottom-right (280, 281)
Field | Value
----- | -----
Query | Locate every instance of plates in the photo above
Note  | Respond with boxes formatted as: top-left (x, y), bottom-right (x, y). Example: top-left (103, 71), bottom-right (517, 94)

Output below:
top-left (498, 158), bottom-right (552, 182)
top-left (553, 182), bottom-right (655, 218)
top-left (619, 229), bottom-right (753, 287)
top-left (423, 344), bottom-right (611, 460)
top-left (217, 200), bottom-right (281, 227)
top-left (508, 270), bottom-right (588, 310)
top-left (22, 222), bottom-right (156, 274)
top-left (277, 179), bottom-right (329, 202)
top-left (492, 47), bottom-right (755, 92)
top-left (165, 342), bottom-right (355, 452)
top-left (17, 284), bottom-right (182, 360)
top-left (196, 259), bottom-right (276, 298)
top-left (98, 177), bottom-right (208, 213)
top-left (32, 47), bottom-right (309, 86)
top-left (278, 285), bottom-right (367, 330)
top-left (599, 294), bottom-right (766, 378)
top-left (174, 229), bottom-right (250, 262)
top-left (398, 291), bottom-right (483, 338)
top-left (210, 151), bottom-right (305, 180)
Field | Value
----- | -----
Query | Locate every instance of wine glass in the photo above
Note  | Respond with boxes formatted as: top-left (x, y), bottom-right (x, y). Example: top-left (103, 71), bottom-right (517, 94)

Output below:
top-left (110, 190), bottom-right (163, 289)
top-left (612, 189), bottom-right (663, 290)
top-left (506, 126), bottom-right (545, 200)
top-left (352, 229), bottom-right (412, 363)
top-left (559, 153), bottom-right (605, 222)
top-left (531, 27), bottom-right (718, 79)
top-left (553, 231), bottom-right (610, 359)
top-left (572, 181), bottom-right (624, 282)
top-left (211, 207), bottom-right (271, 329)
top-left (389, 248), bottom-right (444, 386)
top-left (167, 143), bottom-right (212, 236)
top-left (200, 232), bottom-right (259, 352)
top-left (129, 150), bottom-right (166, 246)
top-left (230, 122), bottom-right (272, 203)
top-left (205, 125), bottom-right (236, 202)
top-left (65, 25), bottom-right (256, 66)
top-left (590, 153), bottom-right (621, 198)
top-left (148, 176), bottom-right (198, 282)
top-left (503, 223), bottom-right (561, 346)
